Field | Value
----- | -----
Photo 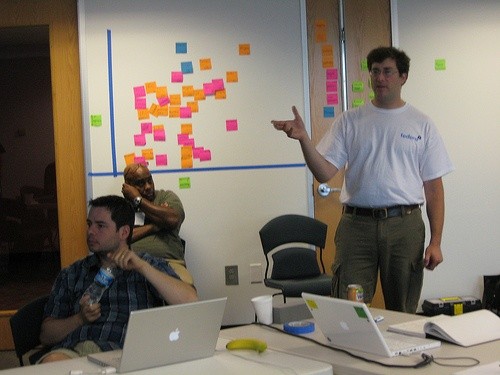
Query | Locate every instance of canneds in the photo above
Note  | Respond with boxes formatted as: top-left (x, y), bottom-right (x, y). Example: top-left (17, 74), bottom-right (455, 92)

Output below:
top-left (346, 284), bottom-right (363, 302)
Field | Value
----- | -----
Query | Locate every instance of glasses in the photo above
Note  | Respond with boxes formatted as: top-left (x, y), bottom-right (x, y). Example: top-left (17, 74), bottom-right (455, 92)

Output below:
top-left (369, 69), bottom-right (399, 77)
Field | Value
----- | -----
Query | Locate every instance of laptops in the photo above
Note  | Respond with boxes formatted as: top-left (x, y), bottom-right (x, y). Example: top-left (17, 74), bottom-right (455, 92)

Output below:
top-left (300, 292), bottom-right (441, 358)
top-left (86, 296), bottom-right (228, 375)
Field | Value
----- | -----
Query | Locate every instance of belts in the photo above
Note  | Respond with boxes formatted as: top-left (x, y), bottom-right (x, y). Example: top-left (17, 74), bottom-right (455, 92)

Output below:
top-left (344, 204), bottom-right (419, 219)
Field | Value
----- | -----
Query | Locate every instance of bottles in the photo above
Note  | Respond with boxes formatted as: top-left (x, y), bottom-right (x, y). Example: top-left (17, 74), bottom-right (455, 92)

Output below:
top-left (82, 255), bottom-right (119, 305)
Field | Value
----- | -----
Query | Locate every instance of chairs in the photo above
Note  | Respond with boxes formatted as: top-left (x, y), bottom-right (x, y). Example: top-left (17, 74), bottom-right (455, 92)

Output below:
top-left (256, 214), bottom-right (334, 322)
top-left (9, 295), bottom-right (51, 367)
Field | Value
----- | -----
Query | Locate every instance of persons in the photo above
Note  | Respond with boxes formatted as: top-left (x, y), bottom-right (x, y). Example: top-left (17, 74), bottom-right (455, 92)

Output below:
top-left (270, 47), bottom-right (451, 316)
top-left (33, 164), bottom-right (196, 364)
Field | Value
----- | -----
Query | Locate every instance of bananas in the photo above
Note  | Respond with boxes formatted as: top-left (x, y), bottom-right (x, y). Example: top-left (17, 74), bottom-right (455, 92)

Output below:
top-left (226, 339), bottom-right (267, 354)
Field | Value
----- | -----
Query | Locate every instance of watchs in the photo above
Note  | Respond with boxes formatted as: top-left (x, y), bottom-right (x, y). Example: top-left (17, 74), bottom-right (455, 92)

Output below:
top-left (134, 196), bottom-right (143, 207)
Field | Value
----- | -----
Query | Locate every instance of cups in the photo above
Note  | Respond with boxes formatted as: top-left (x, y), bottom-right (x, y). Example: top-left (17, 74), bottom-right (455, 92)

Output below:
top-left (250, 294), bottom-right (274, 325)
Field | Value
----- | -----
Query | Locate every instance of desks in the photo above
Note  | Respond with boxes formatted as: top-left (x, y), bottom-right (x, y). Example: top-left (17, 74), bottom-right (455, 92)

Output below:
top-left (218, 308), bottom-right (500, 375)
top-left (0, 336), bottom-right (333, 375)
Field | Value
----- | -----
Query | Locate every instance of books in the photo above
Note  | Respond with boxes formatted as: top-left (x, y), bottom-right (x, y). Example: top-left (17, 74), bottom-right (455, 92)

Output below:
top-left (387, 309), bottom-right (500, 347)
